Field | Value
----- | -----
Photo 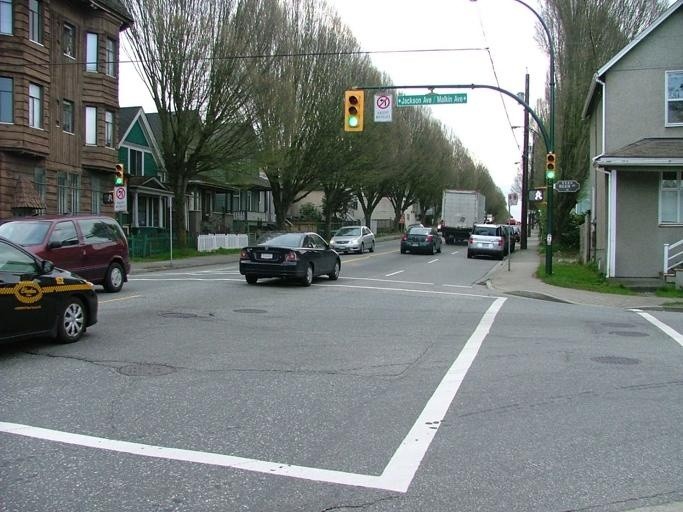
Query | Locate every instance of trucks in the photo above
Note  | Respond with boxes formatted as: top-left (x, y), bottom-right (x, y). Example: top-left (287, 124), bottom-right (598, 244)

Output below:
top-left (441, 188), bottom-right (486, 244)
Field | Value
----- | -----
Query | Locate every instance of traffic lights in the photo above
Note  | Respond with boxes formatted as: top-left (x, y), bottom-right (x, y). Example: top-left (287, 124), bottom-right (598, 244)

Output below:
top-left (545, 153), bottom-right (556, 181)
top-left (114, 162), bottom-right (124, 186)
top-left (345, 90), bottom-right (364, 131)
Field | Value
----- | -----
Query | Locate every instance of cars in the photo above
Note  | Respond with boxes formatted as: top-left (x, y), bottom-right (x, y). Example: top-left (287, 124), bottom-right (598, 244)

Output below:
top-left (468, 224), bottom-right (521, 260)
top-left (0, 237), bottom-right (98, 343)
top-left (239, 226), bottom-right (375, 286)
top-left (400, 223), bottom-right (442, 254)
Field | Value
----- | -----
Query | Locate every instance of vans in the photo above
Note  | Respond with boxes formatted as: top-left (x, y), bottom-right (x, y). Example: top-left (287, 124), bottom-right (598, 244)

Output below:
top-left (0, 213), bottom-right (130, 293)
